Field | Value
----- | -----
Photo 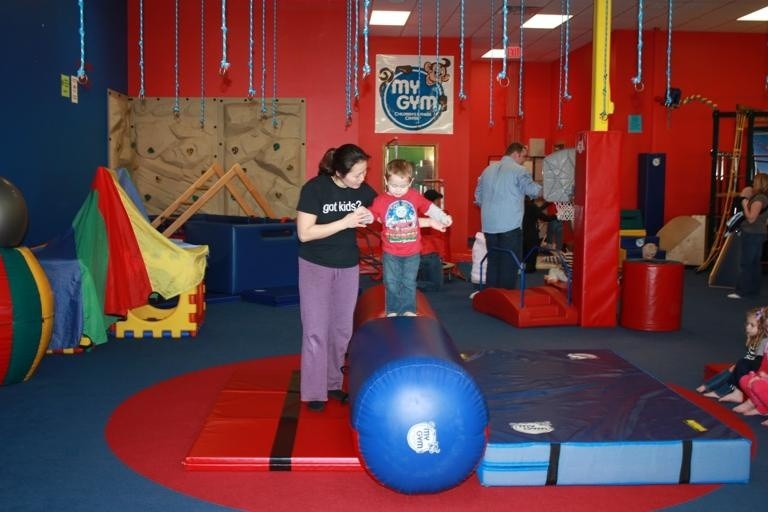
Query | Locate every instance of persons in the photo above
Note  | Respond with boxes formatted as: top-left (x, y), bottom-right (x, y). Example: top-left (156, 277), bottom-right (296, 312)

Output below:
top-left (296, 144), bottom-right (450, 410)
top-left (695, 304), bottom-right (768, 425)
top-left (521, 194), bottom-right (556, 273)
top-left (727, 173), bottom-right (768, 299)
top-left (474, 143), bottom-right (543, 290)
top-left (416, 190), bottom-right (445, 292)
top-left (355, 159), bottom-right (451, 318)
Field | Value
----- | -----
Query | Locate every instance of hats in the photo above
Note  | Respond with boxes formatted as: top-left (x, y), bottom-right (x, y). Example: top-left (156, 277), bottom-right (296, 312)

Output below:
top-left (424, 189), bottom-right (443, 201)
